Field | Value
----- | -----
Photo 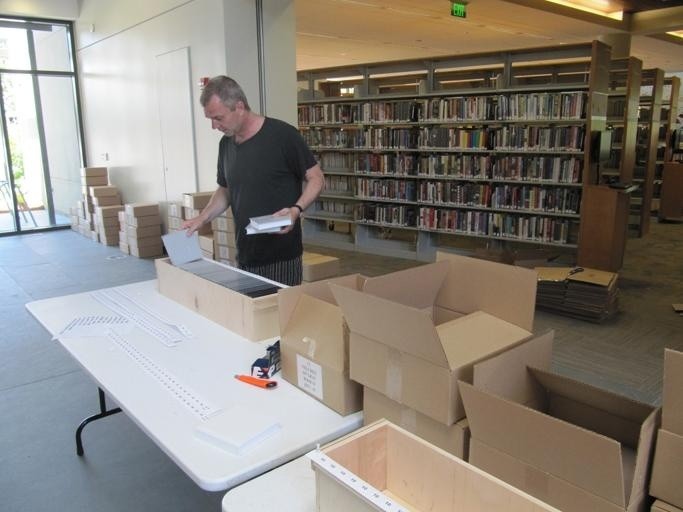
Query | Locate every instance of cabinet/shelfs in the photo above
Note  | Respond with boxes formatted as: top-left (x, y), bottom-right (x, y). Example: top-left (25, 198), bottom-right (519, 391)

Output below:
top-left (297, 40), bottom-right (683, 248)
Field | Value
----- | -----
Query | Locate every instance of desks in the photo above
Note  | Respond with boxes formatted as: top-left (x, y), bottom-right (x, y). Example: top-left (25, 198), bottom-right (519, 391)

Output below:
top-left (24, 279), bottom-right (363, 492)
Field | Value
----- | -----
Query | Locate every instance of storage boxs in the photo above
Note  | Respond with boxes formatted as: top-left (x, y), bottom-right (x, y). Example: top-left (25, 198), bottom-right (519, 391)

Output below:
top-left (276, 272), bottom-right (374, 417)
top-left (71, 167), bottom-right (238, 264)
top-left (647, 347), bottom-right (683, 511)
top-left (327, 250), bottom-right (537, 427)
top-left (454, 326), bottom-right (664, 512)
top-left (360, 385), bottom-right (471, 463)
top-left (154, 254), bottom-right (286, 344)
top-left (310, 417), bottom-right (558, 511)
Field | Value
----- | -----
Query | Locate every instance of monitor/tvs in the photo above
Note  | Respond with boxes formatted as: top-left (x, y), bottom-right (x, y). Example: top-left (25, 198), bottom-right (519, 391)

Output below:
top-left (599, 129), bottom-right (613, 162)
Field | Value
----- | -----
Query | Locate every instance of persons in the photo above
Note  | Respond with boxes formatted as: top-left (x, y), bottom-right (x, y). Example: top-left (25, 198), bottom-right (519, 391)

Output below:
top-left (177, 74), bottom-right (326, 287)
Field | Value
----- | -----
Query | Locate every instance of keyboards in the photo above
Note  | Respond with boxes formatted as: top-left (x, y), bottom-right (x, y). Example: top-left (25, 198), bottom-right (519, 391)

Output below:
top-left (608, 181), bottom-right (633, 189)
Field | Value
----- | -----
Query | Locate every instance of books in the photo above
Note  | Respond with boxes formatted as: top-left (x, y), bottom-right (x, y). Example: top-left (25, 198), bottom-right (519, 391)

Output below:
top-left (160, 212), bottom-right (294, 268)
